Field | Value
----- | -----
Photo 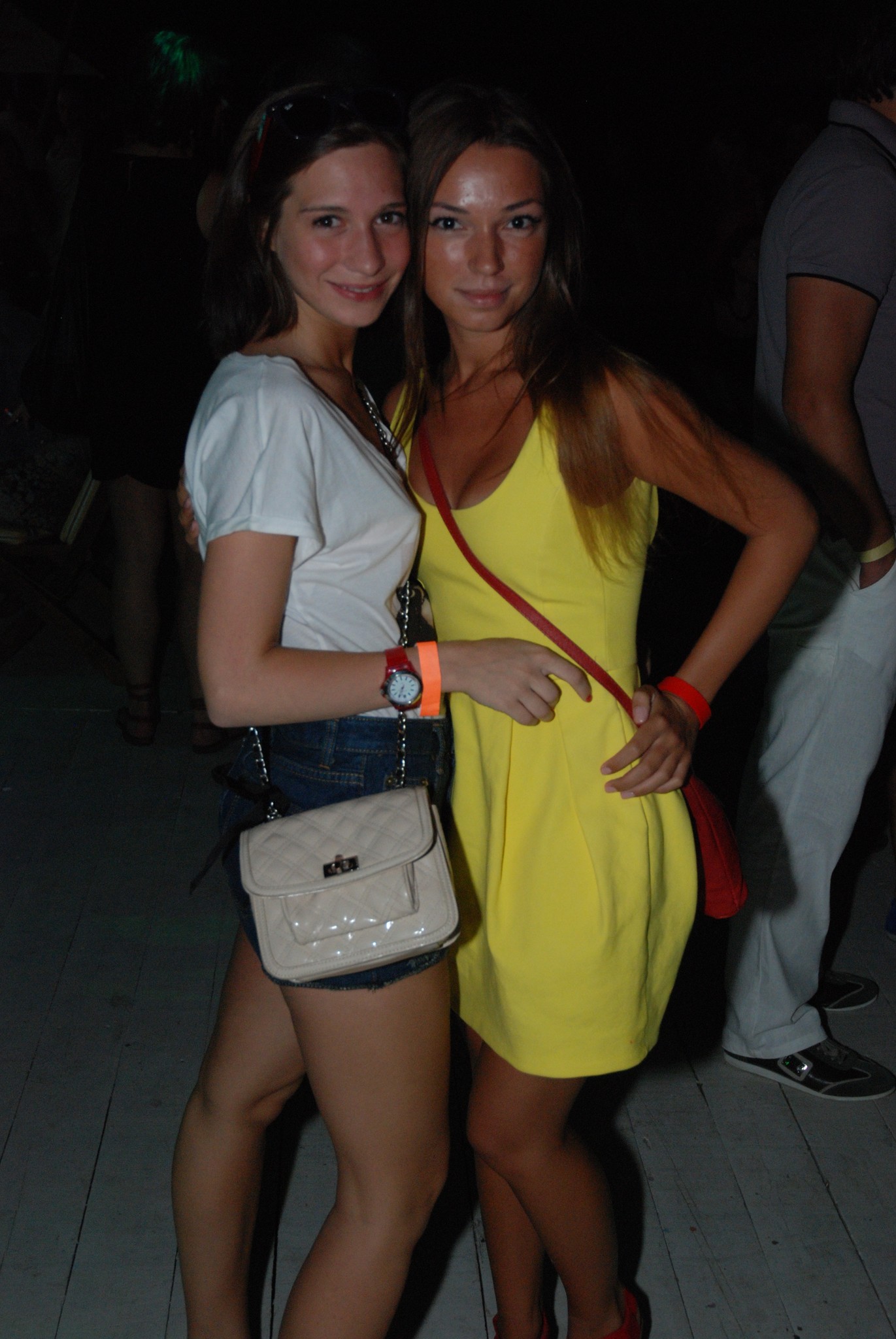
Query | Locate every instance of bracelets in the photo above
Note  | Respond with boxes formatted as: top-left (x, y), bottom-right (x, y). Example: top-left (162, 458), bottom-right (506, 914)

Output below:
top-left (858, 529), bottom-right (896, 563)
top-left (412, 640), bottom-right (442, 719)
top-left (656, 678), bottom-right (712, 733)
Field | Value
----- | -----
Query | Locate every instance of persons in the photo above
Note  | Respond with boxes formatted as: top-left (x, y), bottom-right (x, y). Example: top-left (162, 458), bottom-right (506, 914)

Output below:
top-left (149, 88), bottom-right (590, 1339)
top-left (361, 101), bottom-right (816, 1339)
top-left (80, 31), bottom-right (253, 743)
top-left (724, 2), bottom-right (896, 1109)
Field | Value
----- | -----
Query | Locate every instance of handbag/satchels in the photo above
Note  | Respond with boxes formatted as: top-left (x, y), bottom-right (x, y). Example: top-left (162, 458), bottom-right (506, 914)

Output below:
top-left (241, 782), bottom-right (460, 984)
top-left (677, 773), bottom-right (746, 919)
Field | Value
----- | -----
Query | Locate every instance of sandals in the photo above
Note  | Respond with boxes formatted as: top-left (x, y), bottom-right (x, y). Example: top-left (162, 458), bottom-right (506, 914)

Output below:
top-left (116, 706), bottom-right (158, 745)
top-left (190, 722), bottom-right (251, 754)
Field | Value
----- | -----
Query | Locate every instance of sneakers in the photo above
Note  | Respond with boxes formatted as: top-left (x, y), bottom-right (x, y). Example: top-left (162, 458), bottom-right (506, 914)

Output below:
top-left (805, 970), bottom-right (878, 1011)
top-left (723, 1037), bottom-right (896, 1100)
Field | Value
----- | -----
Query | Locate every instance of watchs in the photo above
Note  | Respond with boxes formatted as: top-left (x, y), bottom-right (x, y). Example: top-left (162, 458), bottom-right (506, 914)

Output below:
top-left (380, 646), bottom-right (422, 713)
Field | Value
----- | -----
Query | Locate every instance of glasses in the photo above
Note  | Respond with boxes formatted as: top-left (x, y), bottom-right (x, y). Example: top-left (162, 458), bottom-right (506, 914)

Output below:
top-left (244, 84), bottom-right (405, 194)
